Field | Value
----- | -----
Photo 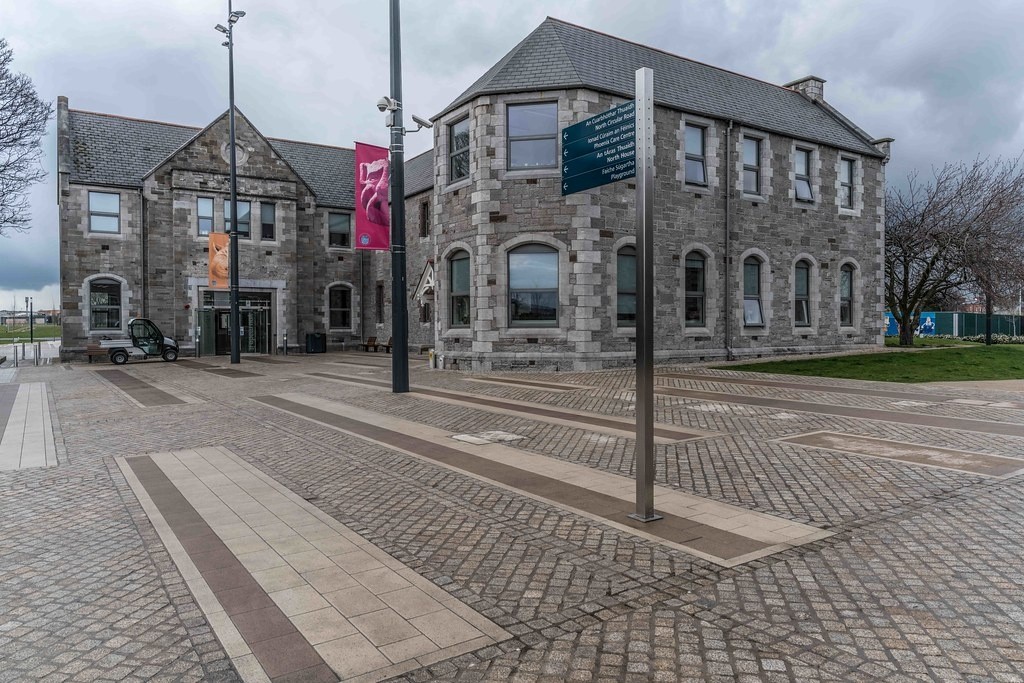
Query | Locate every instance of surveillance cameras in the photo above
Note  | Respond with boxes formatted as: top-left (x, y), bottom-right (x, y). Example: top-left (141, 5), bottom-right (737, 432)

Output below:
top-left (412, 115), bottom-right (433, 129)
top-left (377, 98), bottom-right (388, 112)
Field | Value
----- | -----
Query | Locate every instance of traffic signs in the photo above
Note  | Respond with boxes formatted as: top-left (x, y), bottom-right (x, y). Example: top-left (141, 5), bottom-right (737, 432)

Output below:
top-left (561, 100), bottom-right (637, 196)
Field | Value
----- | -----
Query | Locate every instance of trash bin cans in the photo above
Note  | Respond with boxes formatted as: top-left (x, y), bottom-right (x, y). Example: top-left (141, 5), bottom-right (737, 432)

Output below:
top-left (306, 332), bottom-right (327, 354)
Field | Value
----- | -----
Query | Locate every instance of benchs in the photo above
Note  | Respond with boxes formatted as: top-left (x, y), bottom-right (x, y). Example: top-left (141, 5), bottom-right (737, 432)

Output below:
top-left (418, 344), bottom-right (434, 355)
top-left (86, 344), bottom-right (110, 364)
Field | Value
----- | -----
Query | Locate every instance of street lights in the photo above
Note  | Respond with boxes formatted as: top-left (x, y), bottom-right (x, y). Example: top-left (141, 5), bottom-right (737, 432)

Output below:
top-left (956, 302), bottom-right (966, 311)
top-left (1017, 284), bottom-right (1021, 316)
top-left (214, 10), bottom-right (246, 364)
top-left (975, 296), bottom-right (982, 312)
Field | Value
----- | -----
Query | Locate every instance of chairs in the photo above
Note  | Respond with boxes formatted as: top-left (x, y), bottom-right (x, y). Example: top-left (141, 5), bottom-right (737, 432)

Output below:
top-left (378, 336), bottom-right (392, 353)
top-left (355, 336), bottom-right (378, 353)
